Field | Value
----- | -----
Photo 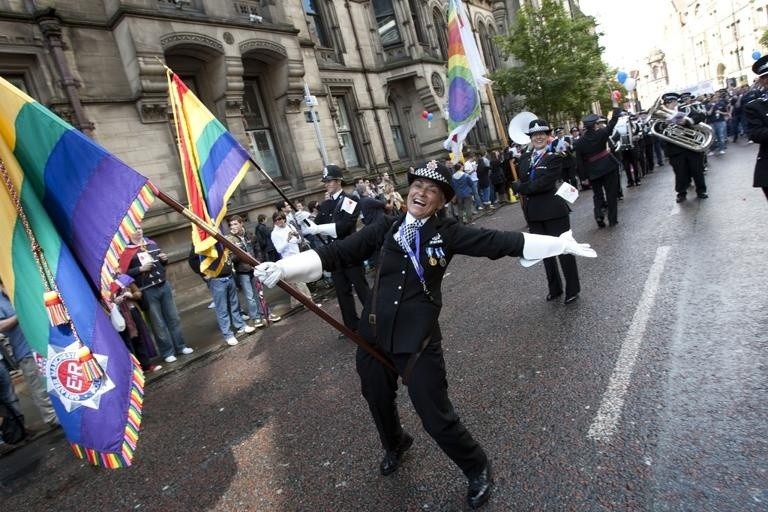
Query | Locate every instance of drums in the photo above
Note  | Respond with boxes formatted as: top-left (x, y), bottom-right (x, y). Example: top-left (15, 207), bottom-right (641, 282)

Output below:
top-left (612, 115), bottom-right (633, 152)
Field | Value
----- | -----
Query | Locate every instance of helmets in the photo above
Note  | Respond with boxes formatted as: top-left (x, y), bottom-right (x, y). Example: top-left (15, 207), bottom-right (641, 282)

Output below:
top-left (320, 165), bottom-right (346, 187)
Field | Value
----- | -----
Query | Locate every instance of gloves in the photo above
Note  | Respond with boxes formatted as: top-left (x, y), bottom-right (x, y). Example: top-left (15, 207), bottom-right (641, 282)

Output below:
top-left (519, 228), bottom-right (597, 267)
top-left (510, 179), bottom-right (521, 196)
top-left (302, 222), bottom-right (338, 239)
top-left (253, 249), bottom-right (323, 289)
top-left (295, 209), bottom-right (311, 226)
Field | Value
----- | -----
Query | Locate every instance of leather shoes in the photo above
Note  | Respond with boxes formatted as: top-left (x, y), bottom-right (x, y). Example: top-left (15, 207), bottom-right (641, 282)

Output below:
top-left (467, 457), bottom-right (491, 509)
top-left (546, 163), bottom-right (709, 305)
top-left (380, 430), bottom-right (413, 475)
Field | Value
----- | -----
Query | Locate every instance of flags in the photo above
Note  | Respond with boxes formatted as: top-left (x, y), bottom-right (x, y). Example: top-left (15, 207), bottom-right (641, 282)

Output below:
top-left (166, 68), bottom-right (250, 278)
top-left (0, 78), bottom-right (159, 469)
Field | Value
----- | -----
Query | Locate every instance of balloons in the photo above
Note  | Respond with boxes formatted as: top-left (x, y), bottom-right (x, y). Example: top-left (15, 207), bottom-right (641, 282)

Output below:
top-left (427, 113), bottom-right (432, 120)
top-left (618, 71), bottom-right (627, 85)
top-left (752, 52), bottom-right (761, 60)
top-left (421, 111), bottom-right (428, 119)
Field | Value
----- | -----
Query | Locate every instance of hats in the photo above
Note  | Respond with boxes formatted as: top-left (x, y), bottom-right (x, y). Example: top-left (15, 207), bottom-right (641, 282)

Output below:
top-left (408, 159), bottom-right (456, 204)
top-left (752, 54), bottom-right (768, 77)
top-left (525, 92), bottom-right (695, 136)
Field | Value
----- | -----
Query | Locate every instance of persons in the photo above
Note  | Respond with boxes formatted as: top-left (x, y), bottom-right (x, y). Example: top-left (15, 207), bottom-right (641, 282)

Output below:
top-left (445, 0), bottom-right (486, 163)
top-left (738, 54), bottom-right (767, 205)
top-left (113, 274), bottom-right (160, 378)
top-left (0, 284), bottom-right (64, 433)
top-left (127, 228), bottom-right (192, 364)
top-left (0, 358), bottom-right (26, 444)
top-left (447, 78), bottom-right (768, 228)
top-left (251, 160), bottom-right (596, 507)
top-left (504, 119), bottom-right (580, 304)
top-left (178, 167), bottom-right (406, 345)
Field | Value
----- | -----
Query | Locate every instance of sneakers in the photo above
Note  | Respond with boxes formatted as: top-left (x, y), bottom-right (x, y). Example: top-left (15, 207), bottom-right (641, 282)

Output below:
top-left (152, 347), bottom-right (194, 372)
top-left (209, 301), bottom-right (323, 346)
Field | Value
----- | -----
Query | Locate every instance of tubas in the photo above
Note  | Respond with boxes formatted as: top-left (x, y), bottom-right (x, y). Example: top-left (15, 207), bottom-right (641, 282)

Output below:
top-left (645, 97), bottom-right (715, 153)
